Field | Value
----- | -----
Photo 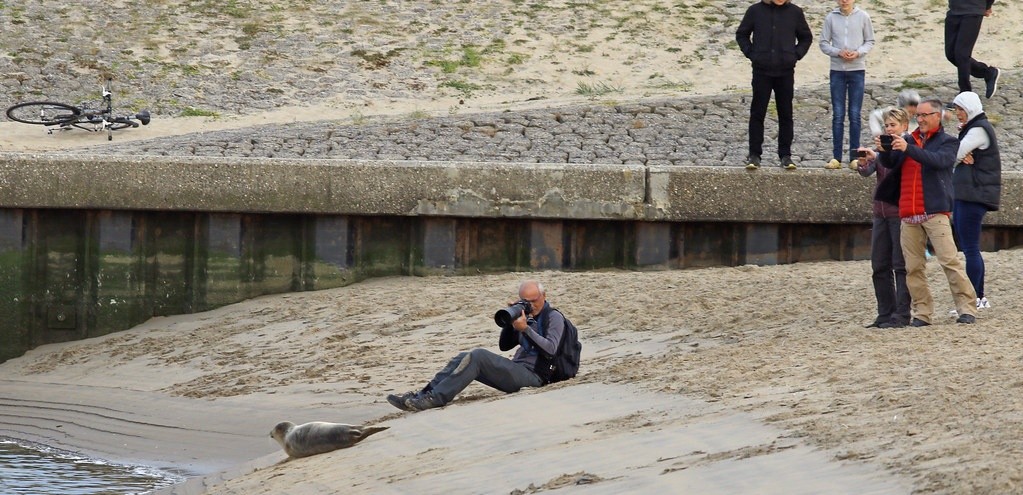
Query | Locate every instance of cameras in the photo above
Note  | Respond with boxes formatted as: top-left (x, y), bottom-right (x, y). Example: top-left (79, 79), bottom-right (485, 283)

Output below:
top-left (494, 300), bottom-right (532, 328)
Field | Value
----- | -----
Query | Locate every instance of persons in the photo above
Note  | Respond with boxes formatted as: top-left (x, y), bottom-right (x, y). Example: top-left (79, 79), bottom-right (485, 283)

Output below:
top-left (856, 90), bottom-right (1001, 329)
top-left (819, 0), bottom-right (875, 169)
top-left (945, 0), bottom-right (1000, 110)
top-left (387, 280), bottom-right (564, 412)
top-left (736, 0), bottom-right (813, 169)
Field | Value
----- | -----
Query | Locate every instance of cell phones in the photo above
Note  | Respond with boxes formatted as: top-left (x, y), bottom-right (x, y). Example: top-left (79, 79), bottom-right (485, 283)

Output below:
top-left (880, 135), bottom-right (896, 151)
top-left (851, 149), bottom-right (867, 157)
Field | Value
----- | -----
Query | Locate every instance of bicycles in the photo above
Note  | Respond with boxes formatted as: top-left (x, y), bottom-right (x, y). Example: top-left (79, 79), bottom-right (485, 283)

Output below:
top-left (6, 77), bottom-right (152, 141)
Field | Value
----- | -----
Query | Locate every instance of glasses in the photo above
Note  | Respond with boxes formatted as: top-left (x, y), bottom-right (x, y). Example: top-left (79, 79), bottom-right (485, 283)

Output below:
top-left (916, 112), bottom-right (937, 117)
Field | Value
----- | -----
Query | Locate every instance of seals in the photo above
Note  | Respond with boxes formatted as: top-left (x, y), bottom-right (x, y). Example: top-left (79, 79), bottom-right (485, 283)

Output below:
top-left (268, 421), bottom-right (391, 465)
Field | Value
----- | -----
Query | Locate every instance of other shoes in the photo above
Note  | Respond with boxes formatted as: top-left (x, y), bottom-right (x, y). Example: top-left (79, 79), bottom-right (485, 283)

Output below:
top-left (825, 158), bottom-right (841, 168)
top-left (956, 313), bottom-right (975, 324)
top-left (866, 321), bottom-right (886, 328)
top-left (981, 297), bottom-right (990, 308)
top-left (879, 317), bottom-right (909, 328)
top-left (848, 159), bottom-right (858, 170)
top-left (909, 318), bottom-right (931, 326)
top-left (950, 297), bottom-right (985, 313)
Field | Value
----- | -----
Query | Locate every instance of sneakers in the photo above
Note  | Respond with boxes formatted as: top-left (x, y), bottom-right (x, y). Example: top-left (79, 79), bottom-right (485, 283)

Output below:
top-left (985, 65), bottom-right (1000, 98)
top-left (746, 156), bottom-right (760, 168)
top-left (781, 156), bottom-right (796, 168)
top-left (404, 393), bottom-right (435, 411)
top-left (386, 391), bottom-right (416, 410)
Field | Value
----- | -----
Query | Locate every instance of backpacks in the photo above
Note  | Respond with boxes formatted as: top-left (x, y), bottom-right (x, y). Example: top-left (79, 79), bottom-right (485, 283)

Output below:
top-left (541, 307), bottom-right (581, 383)
